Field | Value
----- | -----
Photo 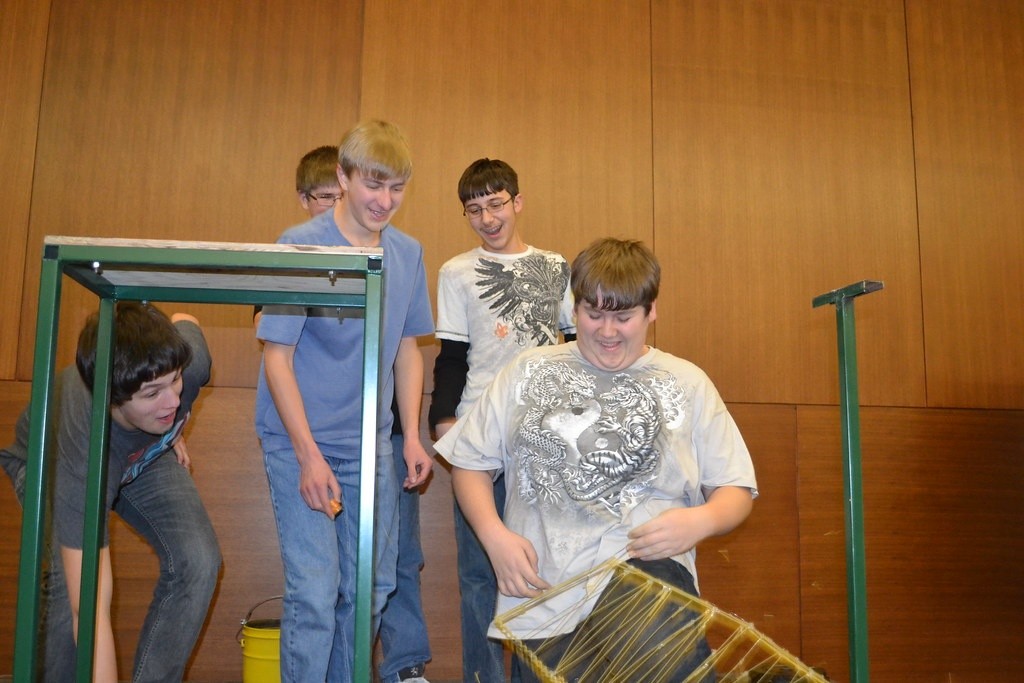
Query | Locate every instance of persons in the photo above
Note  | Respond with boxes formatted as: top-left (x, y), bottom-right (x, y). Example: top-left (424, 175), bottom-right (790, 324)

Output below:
top-left (1, 299), bottom-right (222, 683)
top-left (288, 144), bottom-right (440, 681)
top-left (439, 237), bottom-right (759, 682)
top-left (430, 159), bottom-right (589, 682)
top-left (253, 116), bottom-right (437, 683)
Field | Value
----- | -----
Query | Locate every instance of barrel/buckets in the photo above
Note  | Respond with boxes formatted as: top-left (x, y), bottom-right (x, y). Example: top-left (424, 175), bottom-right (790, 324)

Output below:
top-left (235, 595), bottom-right (284, 683)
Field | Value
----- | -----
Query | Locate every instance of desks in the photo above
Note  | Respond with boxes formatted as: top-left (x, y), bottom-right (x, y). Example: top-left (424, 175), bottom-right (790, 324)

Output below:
top-left (12, 232), bottom-right (386, 683)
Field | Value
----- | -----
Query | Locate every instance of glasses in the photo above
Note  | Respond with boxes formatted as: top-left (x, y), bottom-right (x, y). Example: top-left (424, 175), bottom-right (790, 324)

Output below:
top-left (309, 194), bottom-right (344, 206)
top-left (463, 197), bottom-right (512, 219)
